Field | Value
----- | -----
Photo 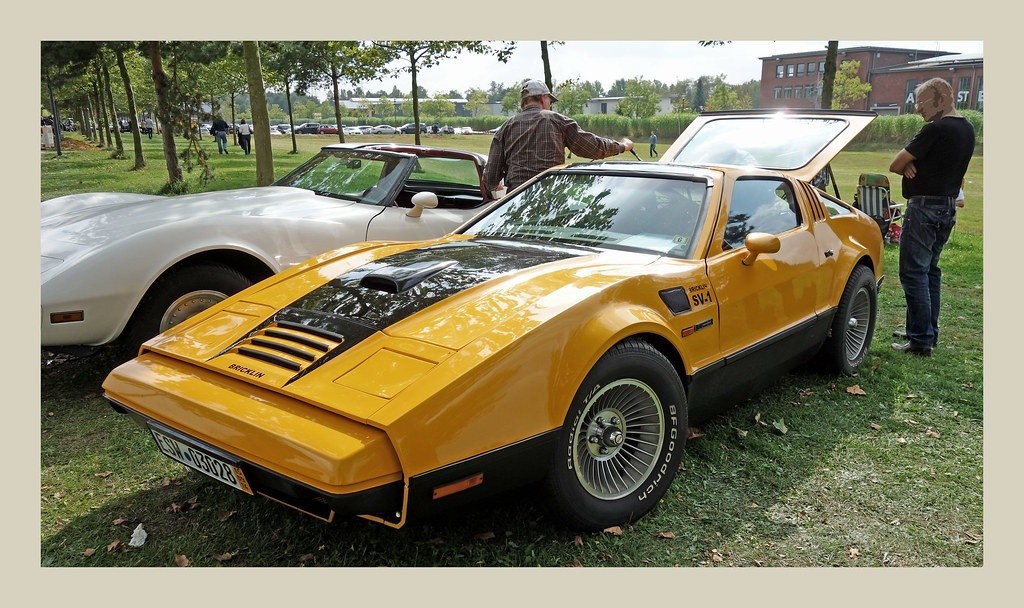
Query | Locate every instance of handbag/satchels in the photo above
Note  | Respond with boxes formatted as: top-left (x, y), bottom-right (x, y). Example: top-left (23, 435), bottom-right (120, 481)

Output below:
top-left (248, 125), bottom-right (254, 134)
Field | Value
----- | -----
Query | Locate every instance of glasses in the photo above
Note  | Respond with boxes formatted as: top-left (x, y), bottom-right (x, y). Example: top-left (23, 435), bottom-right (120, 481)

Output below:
top-left (912, 96), bottom-right (940, 109)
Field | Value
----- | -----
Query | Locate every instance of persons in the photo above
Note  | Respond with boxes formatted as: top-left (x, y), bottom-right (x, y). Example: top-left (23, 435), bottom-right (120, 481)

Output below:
top-left (141, 119), bottom-right (146, 134)
top-left (888, 76), bottom-right (976, 357)
top-left (238, 119), bottom-right (254, 155)
top-left (481, 80), bottom-right (633, 194)
top-left (954, 187), bottom-right (965, 208)
top-left (213, 116), bottom-right (229, 155)
top-left (649, 131), bottom-right (658, 158)
top-left (145, 116), bottom-right (153, 139)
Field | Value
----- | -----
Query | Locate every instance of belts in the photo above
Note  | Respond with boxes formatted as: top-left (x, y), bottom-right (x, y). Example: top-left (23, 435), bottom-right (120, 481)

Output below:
top-left (905, 198), bottom-right (955, 208)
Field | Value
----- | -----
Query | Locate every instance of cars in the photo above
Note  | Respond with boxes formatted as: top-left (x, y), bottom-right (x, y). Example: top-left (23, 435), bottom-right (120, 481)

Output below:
top-left (42, 109), bottom-right (503, 137)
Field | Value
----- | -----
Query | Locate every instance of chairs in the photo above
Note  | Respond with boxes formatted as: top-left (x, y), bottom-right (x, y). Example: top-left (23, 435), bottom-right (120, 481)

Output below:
top-left (855, 173), bottom-right (905, 226)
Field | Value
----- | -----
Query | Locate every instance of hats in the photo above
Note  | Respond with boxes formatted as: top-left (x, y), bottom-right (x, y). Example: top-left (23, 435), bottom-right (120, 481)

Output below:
top-left (521, 79), bottom-right (559, 103)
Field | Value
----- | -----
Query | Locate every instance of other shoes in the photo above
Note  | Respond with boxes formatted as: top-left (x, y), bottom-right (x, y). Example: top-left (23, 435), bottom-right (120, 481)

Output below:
top-left (224, 149), bottom-right (228, 154)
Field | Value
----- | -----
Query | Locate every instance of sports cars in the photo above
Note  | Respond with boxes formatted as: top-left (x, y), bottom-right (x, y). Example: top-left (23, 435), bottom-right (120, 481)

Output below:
top-left (98, 108), bottom-right (889, 537)
top-left (41, 140), bottom-right (507, 373)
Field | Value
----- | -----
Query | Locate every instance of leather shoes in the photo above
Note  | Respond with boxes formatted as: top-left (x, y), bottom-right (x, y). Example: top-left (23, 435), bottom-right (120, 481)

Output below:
top-left (892, 330), bottom-right (907, 340)
top-left (889, 341), bottom-right (932, 358)
